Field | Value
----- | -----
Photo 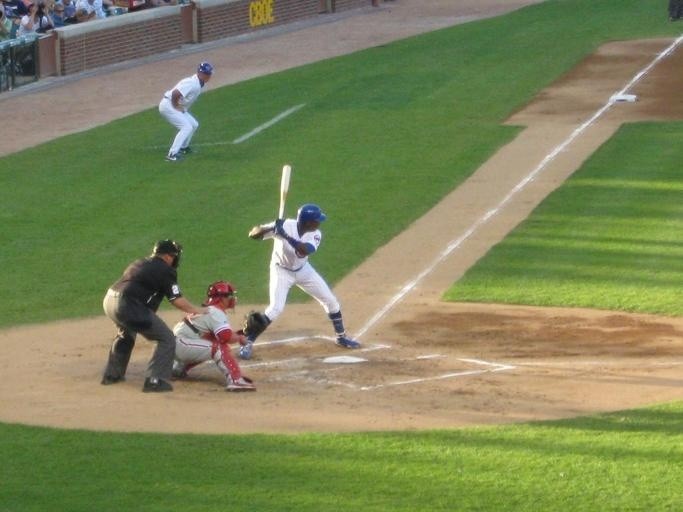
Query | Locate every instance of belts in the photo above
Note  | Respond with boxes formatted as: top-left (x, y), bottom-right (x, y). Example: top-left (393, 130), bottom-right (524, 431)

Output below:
top-left (276, 263), bottom-right (303, 272)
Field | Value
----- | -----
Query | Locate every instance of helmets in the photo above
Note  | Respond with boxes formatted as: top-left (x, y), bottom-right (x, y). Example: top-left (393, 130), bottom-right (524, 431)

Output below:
top-left (152, 238), bottom-right (183, 254)
top-left (207, 281), bottom-right (238, 296)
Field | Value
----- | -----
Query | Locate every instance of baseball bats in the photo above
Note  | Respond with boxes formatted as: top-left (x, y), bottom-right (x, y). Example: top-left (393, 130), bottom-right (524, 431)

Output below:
top-left (275, 165), bottom-right (292, 236)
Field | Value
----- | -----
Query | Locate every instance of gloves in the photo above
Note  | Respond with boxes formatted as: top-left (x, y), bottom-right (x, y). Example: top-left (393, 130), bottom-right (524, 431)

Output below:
top-left (272, 218), bottom-right (288, 239)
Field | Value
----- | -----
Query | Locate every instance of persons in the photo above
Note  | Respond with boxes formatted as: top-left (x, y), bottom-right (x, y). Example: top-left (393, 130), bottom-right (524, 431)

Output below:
top-left (237, 164), bottom-right (362, 361)
top-left (153, 60), bottom-right (213, 163)
top-left (98, 238), bottom-right (209, 396)
top-left (170, 278), bottom-right (257, 393)
top-left (0, 0), bottom-right (195, 44)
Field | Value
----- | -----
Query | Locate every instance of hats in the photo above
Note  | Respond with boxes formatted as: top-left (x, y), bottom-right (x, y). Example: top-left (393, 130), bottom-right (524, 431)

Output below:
top-left (197, 62), bottom-right (212, 75)
top-left (297, 204), bottom-right (326, 225)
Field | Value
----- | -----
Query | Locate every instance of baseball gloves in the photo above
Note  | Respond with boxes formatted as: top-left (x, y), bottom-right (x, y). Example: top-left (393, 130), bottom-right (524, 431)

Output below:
top-left (243, 310), bottom-right (268, 336)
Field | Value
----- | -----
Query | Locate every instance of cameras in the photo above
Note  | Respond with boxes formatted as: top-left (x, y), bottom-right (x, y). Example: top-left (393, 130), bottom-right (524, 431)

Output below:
top-left (35, 5), bottom-right (44, 17)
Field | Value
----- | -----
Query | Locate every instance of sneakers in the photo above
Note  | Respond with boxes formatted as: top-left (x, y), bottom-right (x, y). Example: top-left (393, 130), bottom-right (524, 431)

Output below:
top-left (142, 378), bottom-right (173, 393)
top-left (239, 340), bottom-right (252, 360)
top-left (224, 380), bottom-right (255, 391)
top-left (164, 147), bottom-right (198, 163)
top-left (334, 336), bottom-right (360, 349)
top-left (100, 375), bottom-right (127, 386)
top-left (170, 370), bottom-right (191, 380)
